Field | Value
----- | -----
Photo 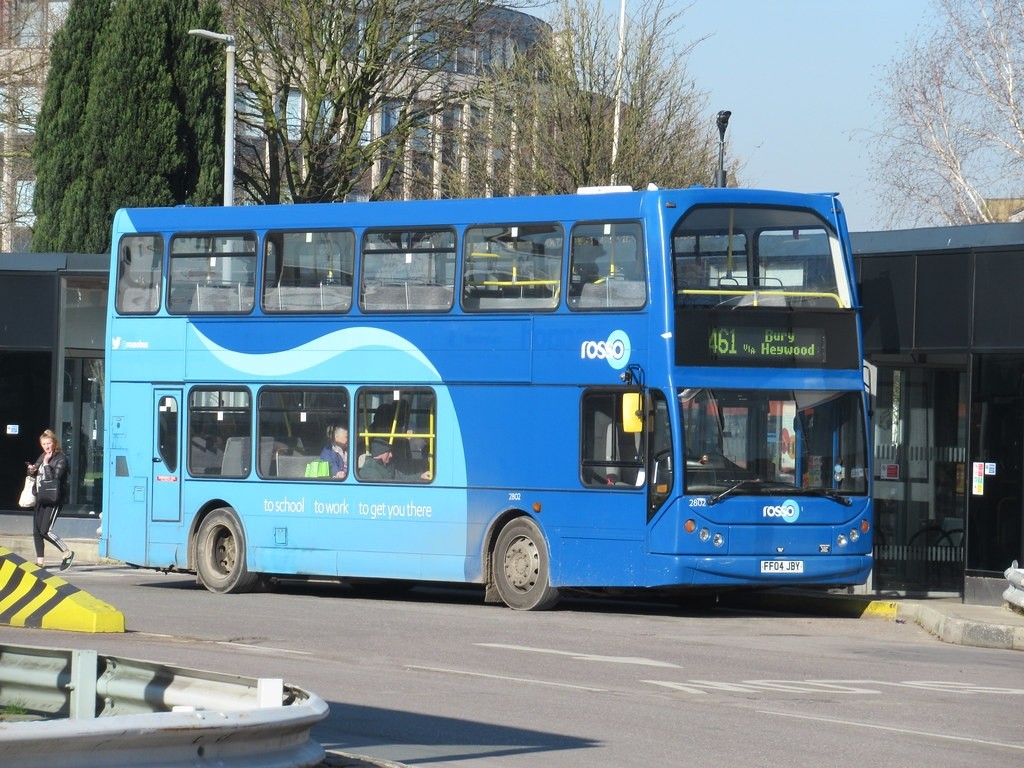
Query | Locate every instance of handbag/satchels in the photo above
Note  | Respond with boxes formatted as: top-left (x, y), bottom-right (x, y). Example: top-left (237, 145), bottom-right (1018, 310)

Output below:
top-left (19, 474), bottom-right (37, 508)
top-left (305, 459), bottom-right (330, 478)
top-left (38, 479), bottom-right (60, 504)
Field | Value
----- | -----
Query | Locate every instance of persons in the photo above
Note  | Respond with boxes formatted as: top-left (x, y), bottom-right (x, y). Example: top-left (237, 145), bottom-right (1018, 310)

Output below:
top-left (18, 429), bottom-right (75, 572)
top-left (318, 421), bottom-right (349, 481)
top-left (361, 395), bottom-right (433, 482)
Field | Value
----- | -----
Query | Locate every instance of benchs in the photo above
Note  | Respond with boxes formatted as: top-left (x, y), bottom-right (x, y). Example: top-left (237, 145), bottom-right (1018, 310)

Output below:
top-left (119, 277), bottom-right (647, 312)
top-left (190, 430), bottom-right (324, 479)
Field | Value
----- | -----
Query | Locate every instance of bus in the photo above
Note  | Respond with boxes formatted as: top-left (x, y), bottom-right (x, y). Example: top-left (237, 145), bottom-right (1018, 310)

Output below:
top-left (97, 182), bottom-right (875, 613)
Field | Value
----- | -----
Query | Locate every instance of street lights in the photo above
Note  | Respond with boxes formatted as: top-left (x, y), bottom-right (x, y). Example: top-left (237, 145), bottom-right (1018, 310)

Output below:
top-left (716, 109), bottom-right (733, 187)
top-left (188, 29), bottom-right (235, 284)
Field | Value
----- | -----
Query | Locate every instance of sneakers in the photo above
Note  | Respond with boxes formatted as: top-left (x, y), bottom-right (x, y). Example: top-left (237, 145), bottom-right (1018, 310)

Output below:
top-left (60, 550), bottom-right (75, 572)
top-left (36, 563), bottom-right (46, 568)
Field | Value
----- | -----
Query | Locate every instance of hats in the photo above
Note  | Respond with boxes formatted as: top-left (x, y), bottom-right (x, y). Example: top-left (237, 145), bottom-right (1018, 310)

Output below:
top-left (371, 439), bottom-right (391, 457)
